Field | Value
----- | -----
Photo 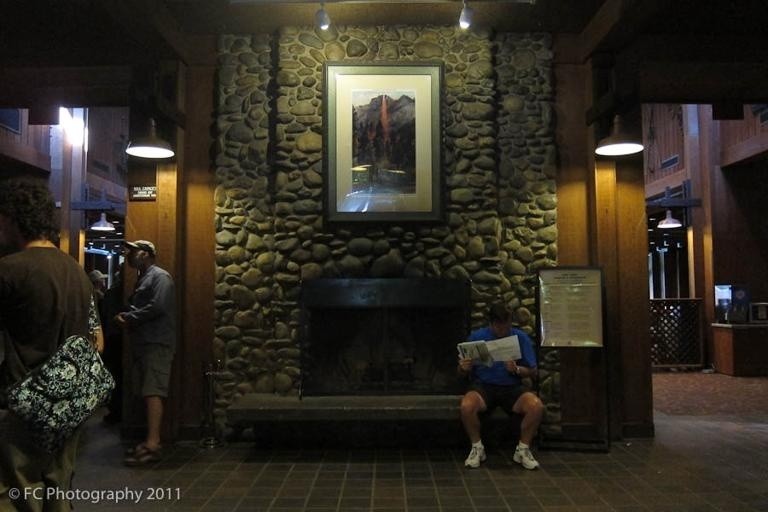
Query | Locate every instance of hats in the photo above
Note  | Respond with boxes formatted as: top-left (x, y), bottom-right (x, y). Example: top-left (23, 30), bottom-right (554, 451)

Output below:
top-left (120, 239), bottom-right (158, 258)
top-left (489, 301), bottom-right (511, 324)
top-left (88, 268), bottom-right (108, 284)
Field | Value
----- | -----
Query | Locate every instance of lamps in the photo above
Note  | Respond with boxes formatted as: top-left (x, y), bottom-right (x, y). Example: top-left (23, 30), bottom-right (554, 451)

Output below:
top-left (459, 0), bottom-right (474, 29)
top-left (125, 114), bottom-right (175, 159)
top-left (91, 213), bottom-right (116, 231)
top-left (656, 210), bottom-right (683, 229)
top-left (594, 108), bottom-right (644, 157)
top-left (315, 0), bottom-right (331, 30)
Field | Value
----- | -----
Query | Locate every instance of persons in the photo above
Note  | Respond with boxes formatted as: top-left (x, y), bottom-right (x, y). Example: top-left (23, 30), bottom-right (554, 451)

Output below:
top-left (87, 262), bottom-right (125, 426)
top-left (111, 239), bottom-right (179, 468)
top-left (1, 177), bottom-right (106, 512)
top-left (459, 304), bottom-right (544, 470)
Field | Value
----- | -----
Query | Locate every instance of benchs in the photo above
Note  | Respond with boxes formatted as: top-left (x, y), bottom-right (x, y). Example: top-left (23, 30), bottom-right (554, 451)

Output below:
top-left (227, 393), bottom-right (543, 412)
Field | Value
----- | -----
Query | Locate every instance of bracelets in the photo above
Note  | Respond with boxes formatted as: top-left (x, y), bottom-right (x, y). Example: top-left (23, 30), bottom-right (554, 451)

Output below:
top-left (515, 366), bottom-right (521, 375)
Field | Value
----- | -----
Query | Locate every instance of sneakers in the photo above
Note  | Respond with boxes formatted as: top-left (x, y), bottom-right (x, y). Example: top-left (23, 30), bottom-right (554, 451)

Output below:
top-left (464, 444), bottom-right (487, 469)
top-left (123, 440), bottom-right (164, 467)
top-left (512, 444), bottom-right (540, 470)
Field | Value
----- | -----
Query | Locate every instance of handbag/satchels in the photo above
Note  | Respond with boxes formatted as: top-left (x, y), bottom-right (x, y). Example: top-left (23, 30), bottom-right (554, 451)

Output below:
top-left (1, 332), bottom-right (117, 452)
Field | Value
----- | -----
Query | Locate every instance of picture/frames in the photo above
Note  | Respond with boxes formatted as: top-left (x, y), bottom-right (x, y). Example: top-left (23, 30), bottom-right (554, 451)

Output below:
top-left (323, 60), bottom-right (443, 223)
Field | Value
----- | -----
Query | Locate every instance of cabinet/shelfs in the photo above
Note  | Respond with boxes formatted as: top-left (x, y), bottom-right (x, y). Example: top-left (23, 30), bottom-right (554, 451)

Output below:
top-left (708, 327), bottom-right (768, 376)
